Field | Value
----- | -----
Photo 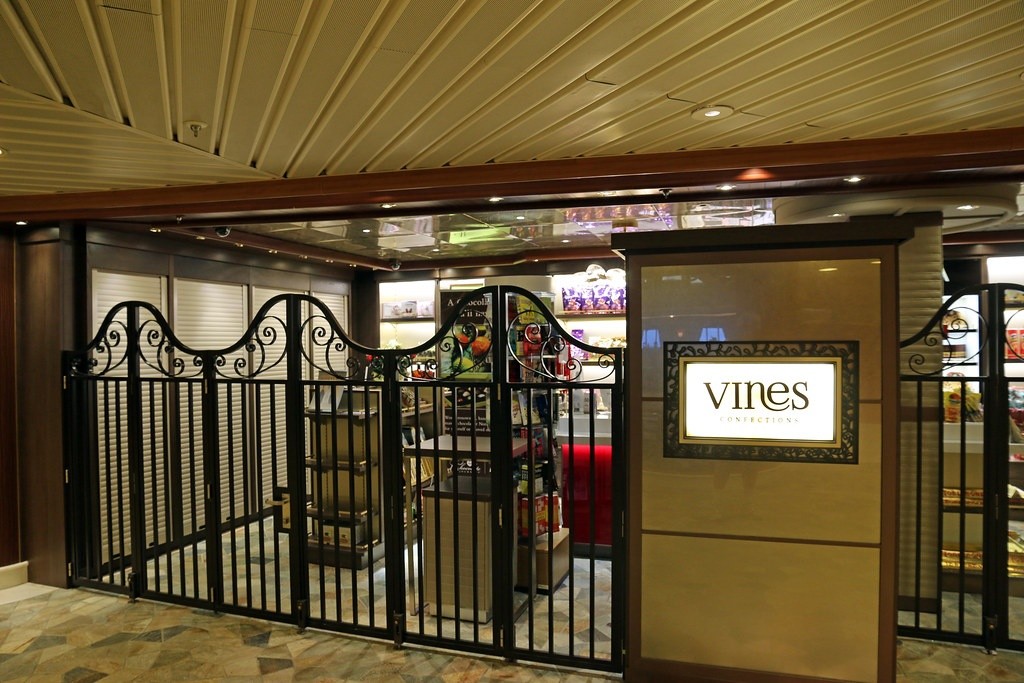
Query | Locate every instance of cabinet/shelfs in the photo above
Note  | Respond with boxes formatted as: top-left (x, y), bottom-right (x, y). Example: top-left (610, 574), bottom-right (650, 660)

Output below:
top-left (379, 279), bottom-right (487, 440)
top-left (942, 254), bottom-right (1024, 597)
top-left (308, 371), bottom-right (443, 571)
top-left (436, 283), bottom-right (569, 596)
top-left (546, 261), bottom-right (626, 436)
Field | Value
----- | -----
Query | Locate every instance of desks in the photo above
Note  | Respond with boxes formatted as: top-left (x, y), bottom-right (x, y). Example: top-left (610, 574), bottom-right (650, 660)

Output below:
top-left (404, 431), bottom-right (537, 625)
